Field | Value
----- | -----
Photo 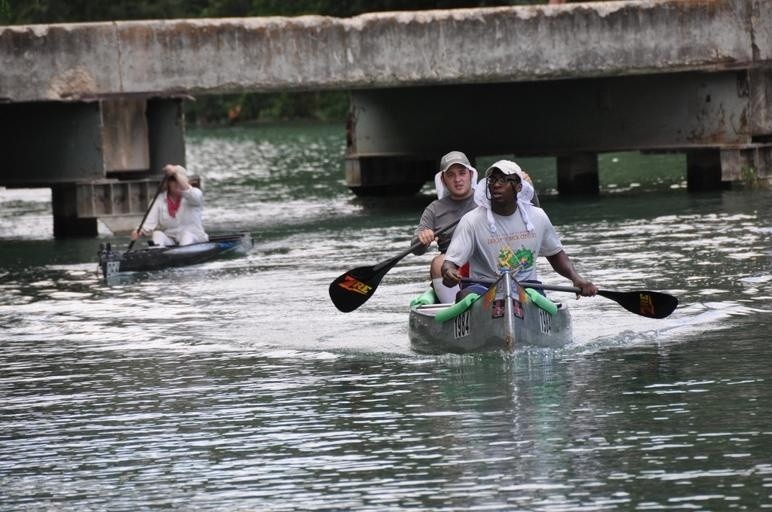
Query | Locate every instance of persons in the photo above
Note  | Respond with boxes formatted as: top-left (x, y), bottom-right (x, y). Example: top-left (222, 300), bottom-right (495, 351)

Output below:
top-left (411, 150), bottom-right (483, 306)
top-left (130, 163), bottom-right (210, 252)
top-left (439, 159), bottom-right (598, 304)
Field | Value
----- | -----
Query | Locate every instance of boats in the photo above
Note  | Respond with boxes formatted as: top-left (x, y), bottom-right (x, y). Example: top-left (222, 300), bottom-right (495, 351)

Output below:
top-left (98, 233), bottom-right (254, 270)
top-left (409, 271), bottom-right (571, 354)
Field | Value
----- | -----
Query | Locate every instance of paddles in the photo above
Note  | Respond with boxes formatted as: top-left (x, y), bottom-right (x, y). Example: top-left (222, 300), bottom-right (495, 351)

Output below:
top-left (161, 242), bottom-right (238, 253)
top-left (329, 218), bottom-right (459, 313)
top-left (461, 277), bottom-right (678, 319)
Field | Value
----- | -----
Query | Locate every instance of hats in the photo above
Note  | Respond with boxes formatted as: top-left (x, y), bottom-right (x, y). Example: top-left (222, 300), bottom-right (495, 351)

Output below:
top-left (485, 159), bottom-right (523, 180)
top-left (440, 151), bottom-right (471, 172)
